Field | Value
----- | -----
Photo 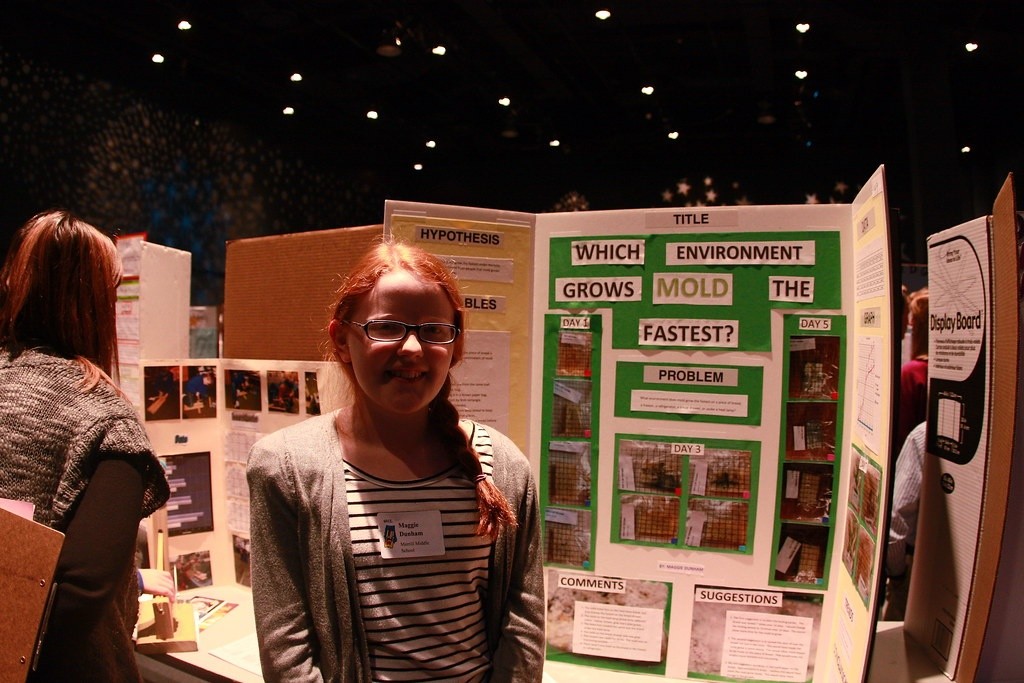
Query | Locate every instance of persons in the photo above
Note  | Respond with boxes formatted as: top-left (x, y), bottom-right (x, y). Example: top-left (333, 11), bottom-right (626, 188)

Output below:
top-left (247, 243), bottom-right (544, 683)
top-left (152, 369), bottom-right (299, 414)
top-left (0, 211), bottom-right (175, 683)
top-left (876, 284), bottom-right (930, 621)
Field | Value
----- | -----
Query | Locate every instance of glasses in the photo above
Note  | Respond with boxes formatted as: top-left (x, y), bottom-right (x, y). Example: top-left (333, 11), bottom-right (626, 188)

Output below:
top-left (341, 319), bottom-right (461, 345)
